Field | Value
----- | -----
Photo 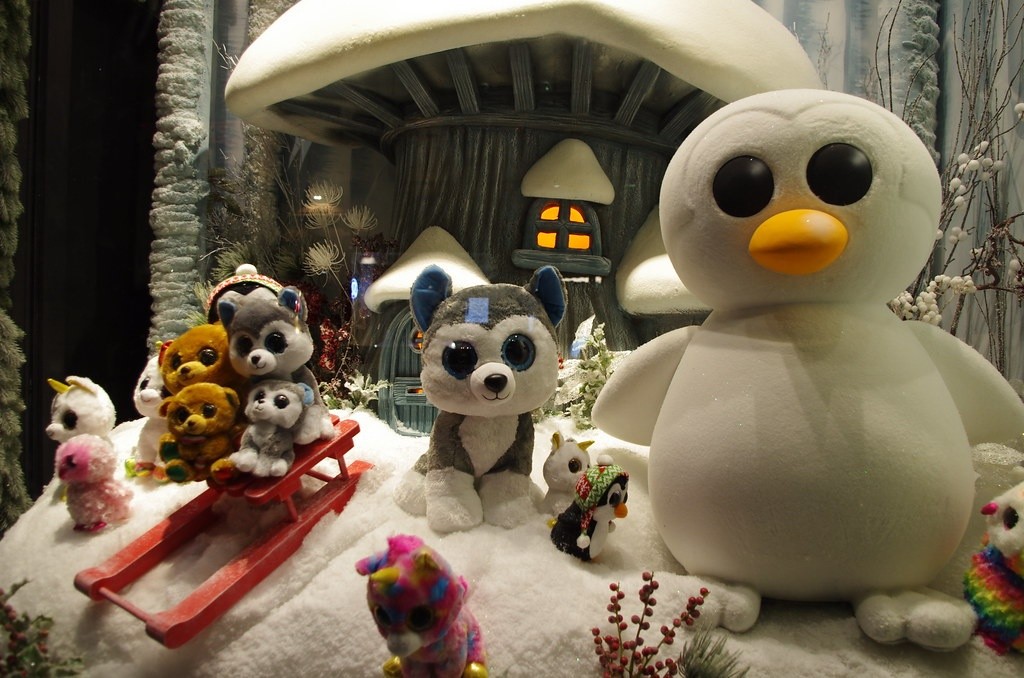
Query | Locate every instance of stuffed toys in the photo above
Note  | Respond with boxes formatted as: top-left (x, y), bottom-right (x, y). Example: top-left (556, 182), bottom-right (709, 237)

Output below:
top-left (356, 535), bottom-right (488, 678)
top-left (591, 88), bottom-right (1024, 657)
top-left (46, 375), bottom-right (133, 534)
top-left (125, 264), bottom-right (341, 515)
top-left (547, 455), bottom-right (630, 563)
top-left (394, 264), bottom-right (568, 533)
top-left (539, 432), bottom-right (594, 517)
